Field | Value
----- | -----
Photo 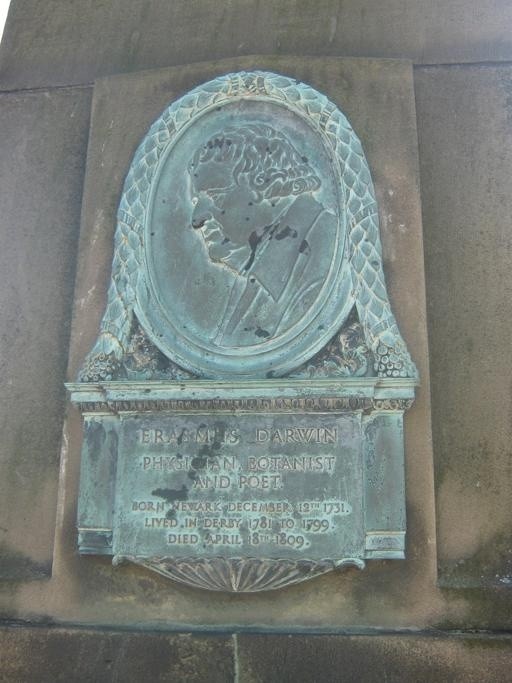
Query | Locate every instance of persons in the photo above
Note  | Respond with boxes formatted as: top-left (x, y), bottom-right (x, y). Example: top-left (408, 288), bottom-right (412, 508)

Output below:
top-left (185, 120), bottom-right (338, 345)
